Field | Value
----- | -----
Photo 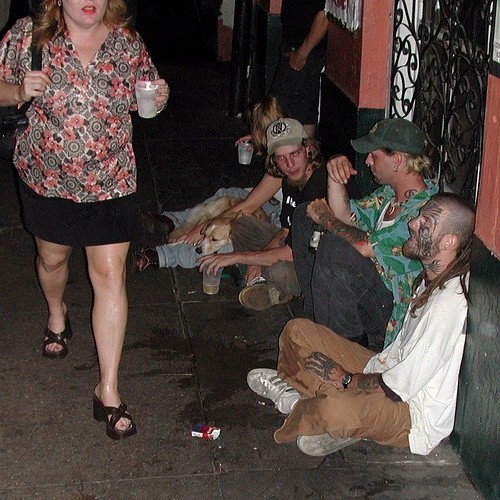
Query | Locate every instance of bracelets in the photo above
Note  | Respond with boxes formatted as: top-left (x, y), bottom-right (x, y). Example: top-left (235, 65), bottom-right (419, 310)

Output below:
top-left (156, 104), bottom-right (165, 114)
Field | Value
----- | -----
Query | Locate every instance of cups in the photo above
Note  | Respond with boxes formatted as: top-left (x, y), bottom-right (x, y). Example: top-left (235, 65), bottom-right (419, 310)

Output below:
top-left (203, 267), bottom-right (223, 295)
top-left (134, 80), bottom-right (160, 118)
top-left (238, 143), bottom-right (253, 164)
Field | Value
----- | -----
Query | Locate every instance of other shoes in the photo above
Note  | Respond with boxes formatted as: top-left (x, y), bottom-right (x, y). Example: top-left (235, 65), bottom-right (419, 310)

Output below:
top-left (130, 247), bottom-right (157, 274)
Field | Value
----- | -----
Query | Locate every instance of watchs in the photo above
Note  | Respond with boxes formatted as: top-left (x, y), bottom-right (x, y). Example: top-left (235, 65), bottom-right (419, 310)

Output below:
top-left (342, 373), bottom-right (353, 389)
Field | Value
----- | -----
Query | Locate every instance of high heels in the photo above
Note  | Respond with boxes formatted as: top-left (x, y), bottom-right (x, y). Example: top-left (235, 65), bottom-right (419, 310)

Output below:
top-left (91, 393), bottom-right (138, 440)
top-left (42, 311), bottom-right (73, 360)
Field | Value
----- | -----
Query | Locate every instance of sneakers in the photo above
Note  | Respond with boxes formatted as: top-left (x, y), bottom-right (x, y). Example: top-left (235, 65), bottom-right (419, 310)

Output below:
top-left (247, 368), bottom-right (301, 413)
top-left (297, 433), bottom-right (363, 457)
top-left (239, 276), bottom-right (294, 309)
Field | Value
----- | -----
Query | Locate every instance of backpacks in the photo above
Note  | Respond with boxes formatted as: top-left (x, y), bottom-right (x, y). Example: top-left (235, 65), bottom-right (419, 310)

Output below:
top-left (0, 12), bottom-right (42, 160)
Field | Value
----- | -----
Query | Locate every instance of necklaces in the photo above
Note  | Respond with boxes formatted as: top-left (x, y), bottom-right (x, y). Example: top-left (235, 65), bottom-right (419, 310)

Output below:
top-left (389, 200), bottom-right (408, 216)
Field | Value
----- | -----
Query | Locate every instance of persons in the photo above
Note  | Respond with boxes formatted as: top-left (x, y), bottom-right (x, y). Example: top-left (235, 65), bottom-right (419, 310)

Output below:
top-left (267, 0), bottom-right (329, 127)
top-left (0, 0), bottom-right (170, 440)
top-left (195, 117), bottom-right (363, 311)
top-left (125, 95), bottom-right (332, 274)
top-left (291, 117), bottom-right (440, 353)
top-left (246, 192), bottom-right (475, 456)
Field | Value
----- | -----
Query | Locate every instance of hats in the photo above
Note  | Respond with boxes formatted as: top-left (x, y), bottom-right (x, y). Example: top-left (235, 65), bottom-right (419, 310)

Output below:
top-left (265, 117), bottom-right (308, 157)
top-left (351, 118), bottom-right (426, 157)
